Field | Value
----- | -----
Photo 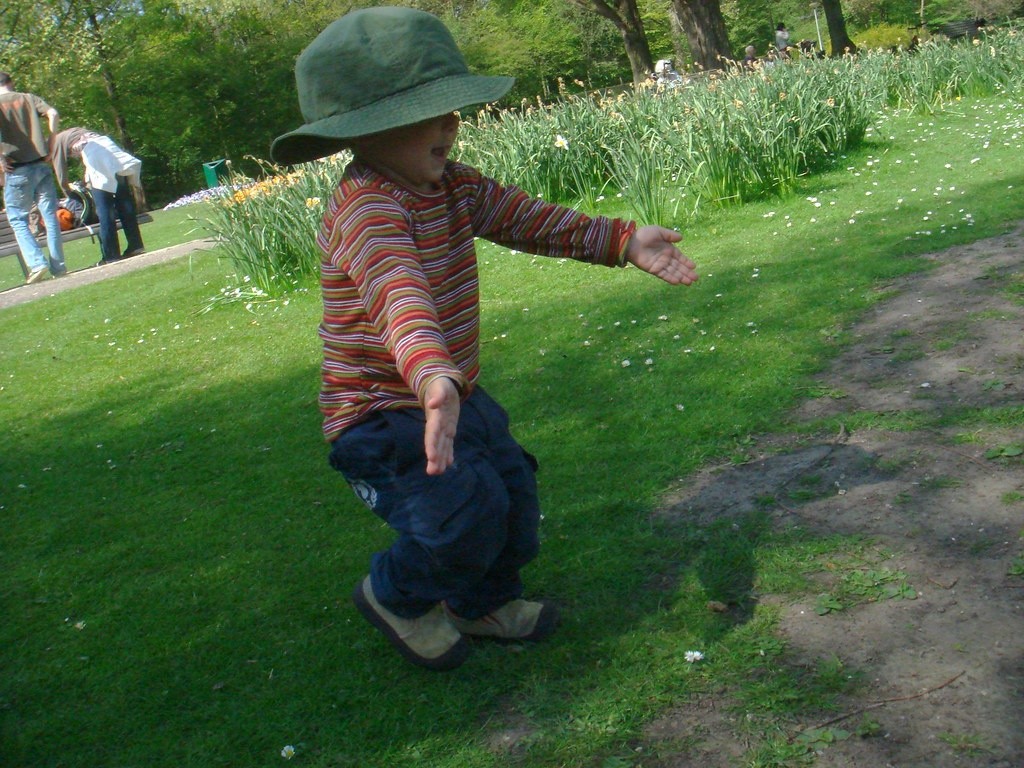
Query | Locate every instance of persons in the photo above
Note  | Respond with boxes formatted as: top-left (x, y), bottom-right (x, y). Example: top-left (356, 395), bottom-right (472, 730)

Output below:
top-left (744, 45), bottom-right (757, 62)
top-left (775, 23), bottom-right (789, 51)
top-left (765, 49), bottom-right (774, 66)
top-left (0, 71), bottom-right (66, 283)
top-left (52, 126), bottom-right (145, 266)
top-left (269, 5), bottom-right (699, 672)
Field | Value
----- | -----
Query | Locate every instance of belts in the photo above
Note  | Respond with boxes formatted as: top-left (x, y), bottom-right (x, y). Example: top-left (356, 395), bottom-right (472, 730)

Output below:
top-left (10, 157), bottom-right (43, 168)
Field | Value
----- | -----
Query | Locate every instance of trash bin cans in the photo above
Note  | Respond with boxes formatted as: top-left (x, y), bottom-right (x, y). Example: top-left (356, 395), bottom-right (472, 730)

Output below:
top-left (64, 190), bottom-right (99, 228)
top-left (202, 159), bottom-right (233, 188)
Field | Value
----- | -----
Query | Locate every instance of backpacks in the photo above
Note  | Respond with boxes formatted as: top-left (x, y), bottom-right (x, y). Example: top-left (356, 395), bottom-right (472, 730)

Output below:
top-left (61, 181), bottom-right (99, 229)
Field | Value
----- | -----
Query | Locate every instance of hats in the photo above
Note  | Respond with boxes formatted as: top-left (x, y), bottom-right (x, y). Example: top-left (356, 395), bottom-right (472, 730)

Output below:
top-left (270, 6), bottom-right (515, 166)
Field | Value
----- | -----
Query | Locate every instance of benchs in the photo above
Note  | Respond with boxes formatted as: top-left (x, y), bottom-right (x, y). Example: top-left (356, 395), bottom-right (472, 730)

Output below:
top-left (0, 210), bottom-right (155, 279)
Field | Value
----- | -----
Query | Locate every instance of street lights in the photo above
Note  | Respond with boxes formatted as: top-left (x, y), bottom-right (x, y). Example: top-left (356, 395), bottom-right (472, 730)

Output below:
top-left (809, 2), bottom-right (823, 52)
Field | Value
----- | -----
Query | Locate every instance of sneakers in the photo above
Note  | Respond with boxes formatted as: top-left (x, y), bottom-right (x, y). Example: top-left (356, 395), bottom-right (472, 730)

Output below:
top-left (353, 574), bottom-right (472, 673)
top-left (441, 599), bottom-right (561, 641)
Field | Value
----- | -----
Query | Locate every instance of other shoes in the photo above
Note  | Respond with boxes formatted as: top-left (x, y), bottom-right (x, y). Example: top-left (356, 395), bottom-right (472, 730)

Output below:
top-left (97, 256), bottom-right (124, 266)
top-left (27, 267), bottom-right (49, 284)
top-left (52, 271), bottom-right (66, 278)
top-left (123, 248), bottom-right (145, 258)
